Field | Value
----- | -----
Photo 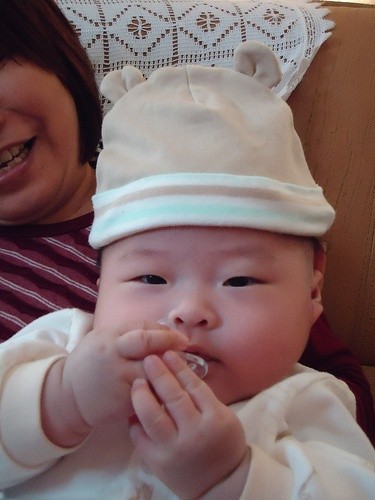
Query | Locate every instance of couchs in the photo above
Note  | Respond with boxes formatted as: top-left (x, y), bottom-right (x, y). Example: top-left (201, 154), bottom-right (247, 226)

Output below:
top-left (44, 0), bottom-right (374, 407)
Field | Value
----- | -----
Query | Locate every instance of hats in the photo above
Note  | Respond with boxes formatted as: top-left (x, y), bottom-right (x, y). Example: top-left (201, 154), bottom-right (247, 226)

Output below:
top-left (87, 40), bottom-right (335, 249)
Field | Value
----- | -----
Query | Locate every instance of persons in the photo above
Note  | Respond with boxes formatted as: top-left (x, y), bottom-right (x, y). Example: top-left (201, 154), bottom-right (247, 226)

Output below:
top-left (0, 0), bottom-right (375, 450)
top-left (1, 40), bottom-right (375, 500)
